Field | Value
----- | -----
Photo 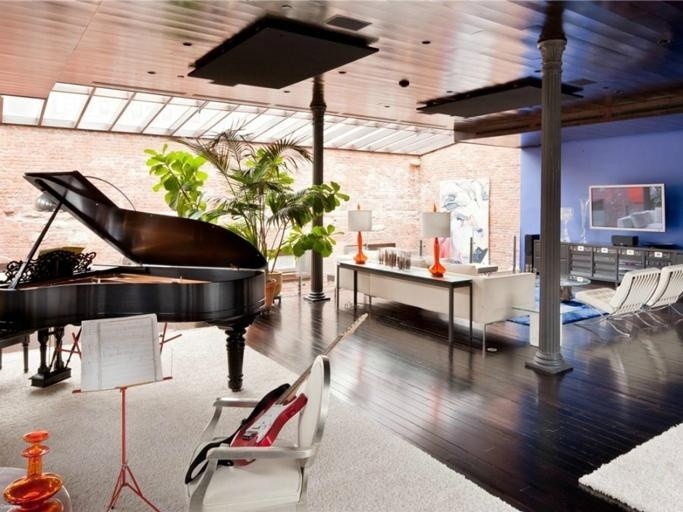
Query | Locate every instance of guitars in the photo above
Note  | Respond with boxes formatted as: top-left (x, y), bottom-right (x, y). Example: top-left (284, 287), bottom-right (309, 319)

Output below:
top-left (228, 314), bottom-right (368, 467)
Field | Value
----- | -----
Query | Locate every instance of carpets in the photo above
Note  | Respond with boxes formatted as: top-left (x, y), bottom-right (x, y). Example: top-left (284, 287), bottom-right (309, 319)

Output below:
top-left (577, 422), bottom-right (683, 512)
top-left (0, 326), bottom-right (523, 512)
top-left (507, 287), bottom-right (609, 326)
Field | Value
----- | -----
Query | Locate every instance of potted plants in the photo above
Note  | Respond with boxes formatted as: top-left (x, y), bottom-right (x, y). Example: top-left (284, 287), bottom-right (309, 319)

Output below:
top-left (143, 119), bottom-right (349, 319)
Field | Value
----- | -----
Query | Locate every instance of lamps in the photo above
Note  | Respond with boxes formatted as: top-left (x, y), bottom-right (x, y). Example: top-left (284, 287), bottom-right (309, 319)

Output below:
top-left (188, 18), bottom-right (379, 90)
top-left (415, 83), bottom-right (584, 118)
top-left (34, 175), bottom-right (136, 211)
top-left (348, 204), bottom-right (372, 265)
top-left (419, 204), bottom-right (451, 277)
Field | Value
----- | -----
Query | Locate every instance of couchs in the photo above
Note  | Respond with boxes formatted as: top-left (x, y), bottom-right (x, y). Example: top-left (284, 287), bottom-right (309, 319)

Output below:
top-left (333, 247), bottom-right (562, 358)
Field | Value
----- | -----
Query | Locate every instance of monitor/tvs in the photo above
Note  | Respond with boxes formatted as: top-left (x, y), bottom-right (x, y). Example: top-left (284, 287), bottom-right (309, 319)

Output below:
top-left (587, 183), bottom-right (668, 236)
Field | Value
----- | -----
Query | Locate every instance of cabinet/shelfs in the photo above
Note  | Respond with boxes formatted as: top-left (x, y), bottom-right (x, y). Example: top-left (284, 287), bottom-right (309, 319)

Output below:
top-left (534, 240), bottom-right (683, 288)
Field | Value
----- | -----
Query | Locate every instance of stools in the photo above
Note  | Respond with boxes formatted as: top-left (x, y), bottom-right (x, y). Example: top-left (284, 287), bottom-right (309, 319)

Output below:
top-left (0, 333), bottom-right (31, 372)
top-left (472, 263), bottom-right (498, 276)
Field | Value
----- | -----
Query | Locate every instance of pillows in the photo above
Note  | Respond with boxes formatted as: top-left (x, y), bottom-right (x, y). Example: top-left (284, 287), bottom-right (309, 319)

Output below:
top-left (407, 257), bottom-right (478, 276)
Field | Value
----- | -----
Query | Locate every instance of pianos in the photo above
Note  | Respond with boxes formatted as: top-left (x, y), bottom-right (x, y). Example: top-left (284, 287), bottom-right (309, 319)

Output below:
top-left (0, 170), bottom-right (268, 392)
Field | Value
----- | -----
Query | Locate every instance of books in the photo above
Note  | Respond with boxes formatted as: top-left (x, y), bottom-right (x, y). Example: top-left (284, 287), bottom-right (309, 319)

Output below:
top-left (80, 313), bottom-right (163, 392)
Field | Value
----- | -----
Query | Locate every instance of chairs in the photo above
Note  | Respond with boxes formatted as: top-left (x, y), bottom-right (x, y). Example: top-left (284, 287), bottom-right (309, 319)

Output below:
top-left (184, 353), bottom-right (331, 512)
top-left (575, 263), bottom-right (683, 338)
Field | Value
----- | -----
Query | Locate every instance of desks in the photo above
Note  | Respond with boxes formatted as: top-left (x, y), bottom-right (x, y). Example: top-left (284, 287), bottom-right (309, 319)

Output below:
top-left (335, 260), bottom-right (474, 345)
